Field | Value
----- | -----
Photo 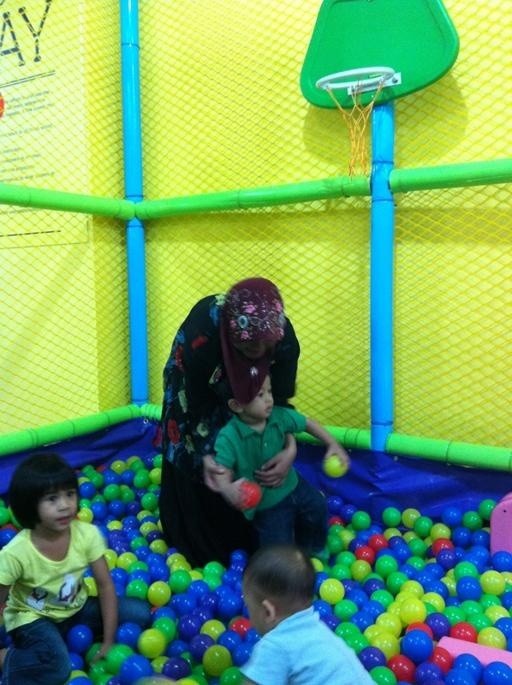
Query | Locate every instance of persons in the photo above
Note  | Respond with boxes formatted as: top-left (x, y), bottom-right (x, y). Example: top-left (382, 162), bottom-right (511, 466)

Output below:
top-left (242, 544), bottom-right (378, 684)
top-left (0, 455), bottom-right (153, 685)
top-left (210, 365), bottom-right (350, 550)
top-left (152, 277), bottom-right (301, 568)
